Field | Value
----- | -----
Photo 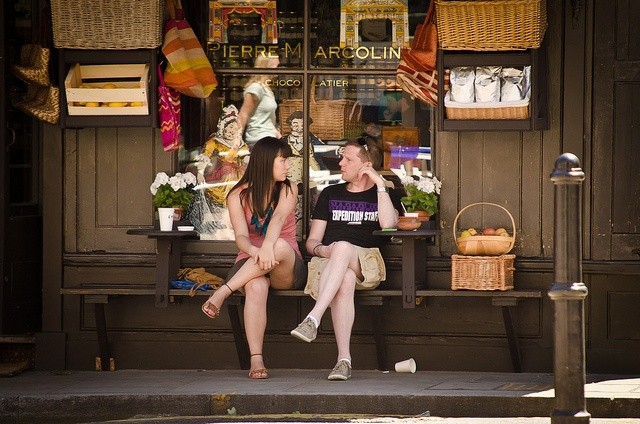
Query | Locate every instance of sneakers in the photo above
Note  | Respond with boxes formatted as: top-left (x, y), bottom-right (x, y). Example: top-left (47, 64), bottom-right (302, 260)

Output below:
top-left (290, 318), bottom-right (317, 342)
top-left (328, 361), bottom-right (351, 381)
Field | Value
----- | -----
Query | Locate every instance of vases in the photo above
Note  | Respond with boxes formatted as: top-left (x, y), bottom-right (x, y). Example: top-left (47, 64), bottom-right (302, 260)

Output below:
top-left (168, 206), bottom-right (184, 221)
top-left (413, 210), bottom-right (430, 221)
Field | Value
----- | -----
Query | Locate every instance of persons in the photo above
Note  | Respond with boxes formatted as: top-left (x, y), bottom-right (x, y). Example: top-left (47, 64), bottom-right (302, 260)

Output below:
top-left (203, 104), bottom-right (251, 185)
top-left (201, 136), bottom-right (308, 380)
top-left (290, 132), bottom-right (401, 380)
top-left (237, 53), bottom-right (280, 143)
top-left (281, 110), bottom-right (325, 183)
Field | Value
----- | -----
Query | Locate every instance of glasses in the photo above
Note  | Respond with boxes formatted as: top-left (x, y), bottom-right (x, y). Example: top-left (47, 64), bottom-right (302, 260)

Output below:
top-left (357, 137), bottom-right (372, 163)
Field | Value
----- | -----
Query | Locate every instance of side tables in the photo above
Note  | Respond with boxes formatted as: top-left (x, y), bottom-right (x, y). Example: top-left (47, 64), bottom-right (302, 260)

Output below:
top-left (127, 228), bottom-right (200, 309)
top-left (373, 227), bottom-right (439, 310)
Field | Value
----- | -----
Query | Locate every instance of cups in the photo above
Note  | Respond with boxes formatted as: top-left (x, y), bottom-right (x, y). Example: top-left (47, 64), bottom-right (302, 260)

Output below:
top-left (403, 211), bottom-right (420, 232)
top-left (393, 357), bottom-right (418, 374)
top-left (156, 204), bottom-right (176, 233)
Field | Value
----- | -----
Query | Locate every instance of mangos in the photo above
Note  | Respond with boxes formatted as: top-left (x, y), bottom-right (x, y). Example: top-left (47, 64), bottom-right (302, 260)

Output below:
top-left (76, 84), bottom-right (142, 107)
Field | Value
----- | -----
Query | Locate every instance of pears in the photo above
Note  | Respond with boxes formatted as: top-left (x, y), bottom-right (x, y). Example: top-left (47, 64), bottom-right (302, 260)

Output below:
top-left (461, 231), bottom-right (471, 236)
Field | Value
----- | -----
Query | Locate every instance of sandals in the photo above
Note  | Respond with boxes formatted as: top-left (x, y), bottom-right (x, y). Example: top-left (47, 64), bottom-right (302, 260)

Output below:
top-left (249, 366), bottom-right (268, 379)
top-left (202, 292), bottom-right (220, 318)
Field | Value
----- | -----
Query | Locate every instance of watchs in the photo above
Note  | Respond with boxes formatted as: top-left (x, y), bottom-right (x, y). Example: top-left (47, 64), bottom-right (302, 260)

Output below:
top-left (377, 186), bottom-right (389, 192)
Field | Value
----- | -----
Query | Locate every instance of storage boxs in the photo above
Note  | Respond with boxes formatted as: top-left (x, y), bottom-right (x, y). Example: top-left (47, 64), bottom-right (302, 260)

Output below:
top-left (56, 44), bottom-right (161, 130)
top-left (436, 46), bottom-right (550, 133)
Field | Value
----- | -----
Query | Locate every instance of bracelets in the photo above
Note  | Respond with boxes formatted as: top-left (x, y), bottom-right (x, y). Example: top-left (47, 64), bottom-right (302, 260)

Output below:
top-left (313, 244), bottom-right (323, 256)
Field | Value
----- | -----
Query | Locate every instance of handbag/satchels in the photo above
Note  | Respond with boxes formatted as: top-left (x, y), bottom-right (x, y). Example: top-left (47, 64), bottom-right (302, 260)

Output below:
top-left (397, 60), bottom-right (449, 107)
top-left (10, 3), bottom-right (50, 87)
top-left (399, 1), bottom-right (435, 69)
top-left (14, 54), bottom-right (61, 124)
top-left (186, 268), bottom-right (224, 297)
top-left (162, 0), bottom-right (218, 98)
top-left (157, 65), bottom-right (181, 152)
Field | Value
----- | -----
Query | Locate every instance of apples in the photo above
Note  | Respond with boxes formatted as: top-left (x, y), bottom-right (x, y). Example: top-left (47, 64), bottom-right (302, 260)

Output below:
top-left (500, 232), bottom-right (510, 237)
top-left (495, 228), bottom-right (506, 235)
top-left (482, 228), bottom-right (496, 236)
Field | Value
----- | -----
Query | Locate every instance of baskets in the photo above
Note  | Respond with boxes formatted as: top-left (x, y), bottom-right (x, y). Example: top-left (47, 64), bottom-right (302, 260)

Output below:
top-left (444, 91), bottom-right (531, 120)
top-left (453, 202), bottom-right (516, 255)
top-left (280, 75), bottom-right (362, 140)
top-left (51, 0), bottom-right (162, 49)
top-left (451, 254), bottom-right (516, 292)
top-left (435, 0), bottom-right (549, 50)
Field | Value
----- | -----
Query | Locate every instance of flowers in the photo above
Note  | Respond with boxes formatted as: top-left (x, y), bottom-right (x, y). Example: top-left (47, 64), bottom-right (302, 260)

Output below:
top-left (400, 175), bottom-right (442, 216)
top-left (148, 170), bottom-right (198, 209)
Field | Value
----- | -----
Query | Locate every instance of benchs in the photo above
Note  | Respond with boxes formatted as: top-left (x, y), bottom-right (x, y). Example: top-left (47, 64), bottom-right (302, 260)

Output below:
top-left (60, 283), bottom-right (540, 373)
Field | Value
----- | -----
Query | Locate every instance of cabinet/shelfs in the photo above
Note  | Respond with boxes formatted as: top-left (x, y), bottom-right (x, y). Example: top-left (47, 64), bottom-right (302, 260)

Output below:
top-left (198, 0), bottom-right (417, 145)
